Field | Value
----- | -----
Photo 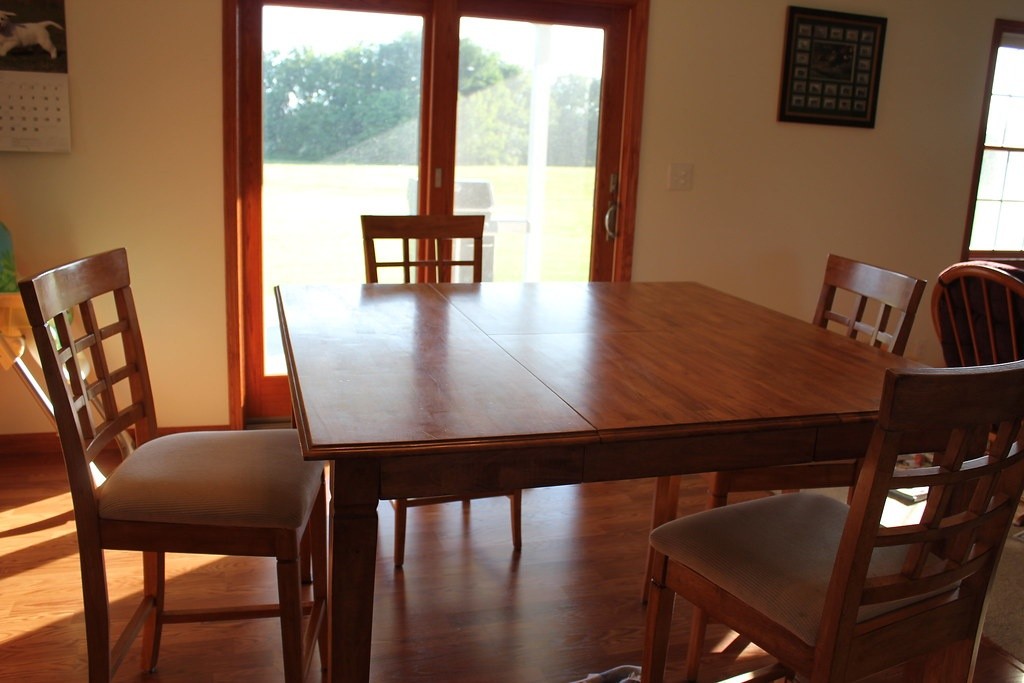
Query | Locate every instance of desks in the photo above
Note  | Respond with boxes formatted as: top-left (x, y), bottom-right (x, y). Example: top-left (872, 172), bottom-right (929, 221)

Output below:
top-left (274, 281), bottom-right (992, 683)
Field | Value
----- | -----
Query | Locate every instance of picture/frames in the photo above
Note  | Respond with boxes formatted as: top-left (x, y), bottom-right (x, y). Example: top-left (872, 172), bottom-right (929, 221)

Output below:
top-left (776, 5), bottom-right (888, 129)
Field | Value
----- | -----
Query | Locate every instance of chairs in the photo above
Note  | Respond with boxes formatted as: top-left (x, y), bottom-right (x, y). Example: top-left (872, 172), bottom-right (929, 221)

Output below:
top-left (361, 215), bottom-right (523, 568)
top-left (641, 253), bottom-right (928, 606)
top-left (930, 261), bottom-right (1024, 559)
top-left (641, 360), bottom-right (1024, 683)
top-left (18, 246), bottom-right (329, 683)
